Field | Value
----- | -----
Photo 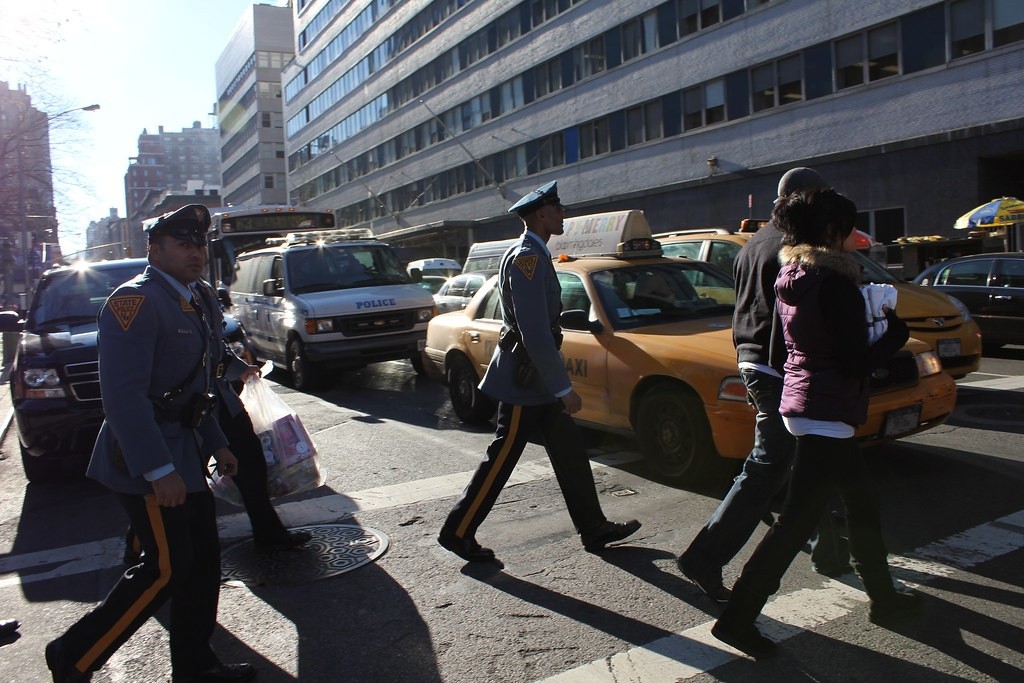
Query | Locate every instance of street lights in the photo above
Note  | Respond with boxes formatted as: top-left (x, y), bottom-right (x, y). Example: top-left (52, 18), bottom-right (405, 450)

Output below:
top-left (14, 103), bottom-right (101, 312)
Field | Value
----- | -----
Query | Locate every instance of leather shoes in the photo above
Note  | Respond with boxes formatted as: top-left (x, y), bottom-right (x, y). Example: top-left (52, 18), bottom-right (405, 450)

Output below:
top-left (45, 640), bottom-right (90, 683)
top-left (1, 618), bottom-right (21, 637)
top-left (171, 662), bottom-right (254, 682)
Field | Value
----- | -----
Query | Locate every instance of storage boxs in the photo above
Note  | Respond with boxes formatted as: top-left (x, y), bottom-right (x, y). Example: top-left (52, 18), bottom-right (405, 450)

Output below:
top-left (257, 414), bottom-right (320, 501)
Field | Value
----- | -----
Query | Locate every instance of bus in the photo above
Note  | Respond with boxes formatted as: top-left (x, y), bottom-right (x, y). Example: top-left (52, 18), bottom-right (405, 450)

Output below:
top-left (200, 202), bottom-right (338, 294)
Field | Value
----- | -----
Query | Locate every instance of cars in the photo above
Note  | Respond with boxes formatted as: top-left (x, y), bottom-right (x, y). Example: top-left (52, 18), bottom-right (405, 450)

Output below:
top-left (431, 268), bottom-right (502, 314)
top-left (421, 207), bottom-right (959, 487)
top-left (909, 250), bottom-right (1024, 350)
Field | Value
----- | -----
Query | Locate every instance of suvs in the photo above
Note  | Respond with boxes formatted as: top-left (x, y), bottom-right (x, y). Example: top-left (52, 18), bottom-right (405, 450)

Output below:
top-left (563, 226), bottom-right (984, 379)
top-left (0, 256), bottom-right (258, 486)
top-left (226, 227), bottom-right (440, 393)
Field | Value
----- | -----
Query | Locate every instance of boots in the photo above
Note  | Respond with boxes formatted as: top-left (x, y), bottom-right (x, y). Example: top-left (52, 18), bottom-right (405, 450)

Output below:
top-left (711, 578), bottom-right (779, 658)
top-left (855, 563), bottom-right (921, 624)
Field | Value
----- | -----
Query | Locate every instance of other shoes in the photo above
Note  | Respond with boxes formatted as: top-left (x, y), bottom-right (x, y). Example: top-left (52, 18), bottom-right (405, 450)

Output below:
top-left (581, 519), bottom-right (641, 550)
top-left (255, 530), bottom-right (310, 550)
top-left (814, 542), bottom-right (850, 575)
top-left (675, 558), bottom-right (731, 603)
top-left (438, 530), bottom-right (496, 562)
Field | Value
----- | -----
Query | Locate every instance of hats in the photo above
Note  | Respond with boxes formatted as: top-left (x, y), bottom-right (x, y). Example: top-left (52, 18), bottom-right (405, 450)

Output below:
top-left (508, 181), bottom-right (566, 218)
top-left (777, 167), bottom-right (827, 197)
top-left (143, 204), bottom-right (211, 246)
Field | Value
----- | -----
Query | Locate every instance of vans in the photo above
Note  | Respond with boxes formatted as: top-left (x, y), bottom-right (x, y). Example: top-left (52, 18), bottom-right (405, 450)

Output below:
top-left (461, 238), bottom-right (521, 272)
top-left (404, 257), bottom-right (462, 297)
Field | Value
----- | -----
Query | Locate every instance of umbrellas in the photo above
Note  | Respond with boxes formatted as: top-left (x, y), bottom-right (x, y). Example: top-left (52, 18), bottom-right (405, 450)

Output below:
top-left (952, 196), bottom-right (1024, 252)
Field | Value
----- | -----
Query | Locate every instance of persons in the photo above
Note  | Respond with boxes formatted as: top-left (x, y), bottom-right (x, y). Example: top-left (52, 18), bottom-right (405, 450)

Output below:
top-left (437, 180), bottom-right (642, 563)
top-left (711, 184), bottom-right (923, 658)
top-left (46, 204), bottom-right (259, 682)
top-left (122, 273), bottom-right (313, 567)
top-left (675, 166), bottom-right (854, 603)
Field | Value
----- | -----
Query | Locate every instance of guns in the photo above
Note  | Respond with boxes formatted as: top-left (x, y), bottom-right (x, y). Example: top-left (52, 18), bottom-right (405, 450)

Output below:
top-left (112, 439), bottom-right (126, 469)
top-left (498, 329), bottom-right (525, 355)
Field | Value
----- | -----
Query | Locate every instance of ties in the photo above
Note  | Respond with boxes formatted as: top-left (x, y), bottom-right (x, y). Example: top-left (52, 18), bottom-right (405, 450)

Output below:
top-left (189, 296), bottom-right (203, 320)
